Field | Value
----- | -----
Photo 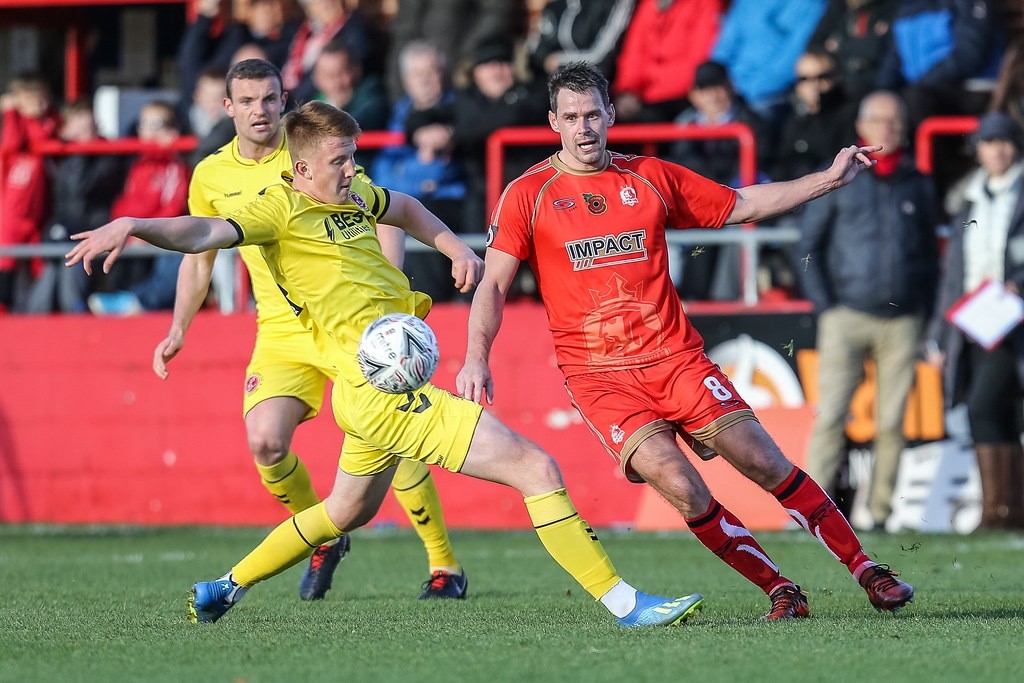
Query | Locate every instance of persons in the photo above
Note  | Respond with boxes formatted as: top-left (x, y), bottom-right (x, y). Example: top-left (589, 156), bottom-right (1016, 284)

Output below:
top-left (918, 114), bottom-right (1024, 528)
top-left (0, 0), bottom-right (1005, 314)
top-left (66, 102), bottom-right (708, 624)
top-left (152, 60), bottom-right (471, 632)
top-left (455, 58), bottom-right (915, 620)
top-left (794, 91), bottom-right (942, 529)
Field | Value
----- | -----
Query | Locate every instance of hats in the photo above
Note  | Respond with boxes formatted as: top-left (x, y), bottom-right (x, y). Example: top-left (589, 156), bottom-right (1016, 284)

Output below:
top-left (978, 114), bottom-right (1021, 142)
top-left (472, 37), bottom-right (513, 66)
top-left (695, 61), bottom-right (726, 88)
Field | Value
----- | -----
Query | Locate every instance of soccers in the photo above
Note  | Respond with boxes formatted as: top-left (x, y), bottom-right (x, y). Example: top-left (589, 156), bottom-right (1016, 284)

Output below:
top-left (357, 312), bottom-right (440, 394)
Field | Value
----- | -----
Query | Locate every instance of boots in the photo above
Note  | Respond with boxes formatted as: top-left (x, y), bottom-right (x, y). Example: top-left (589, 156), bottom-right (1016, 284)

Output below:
top-left (970, 441), bottom-right (1023, 534)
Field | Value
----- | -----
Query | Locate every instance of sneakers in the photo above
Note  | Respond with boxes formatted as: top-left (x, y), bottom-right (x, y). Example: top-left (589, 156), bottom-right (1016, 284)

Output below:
top-left (615, 591), bottom-right (707, 628)
top-left (760, 584), bottom-right (809, 622)
top-left (417, 563), bottom-right (468, 600)
top-left (186, 577), bottom-right (234, 625)
top-left (859, 563), bottom-right (913, 612)
top-left (299, 532), bottom-right (351, 600)
